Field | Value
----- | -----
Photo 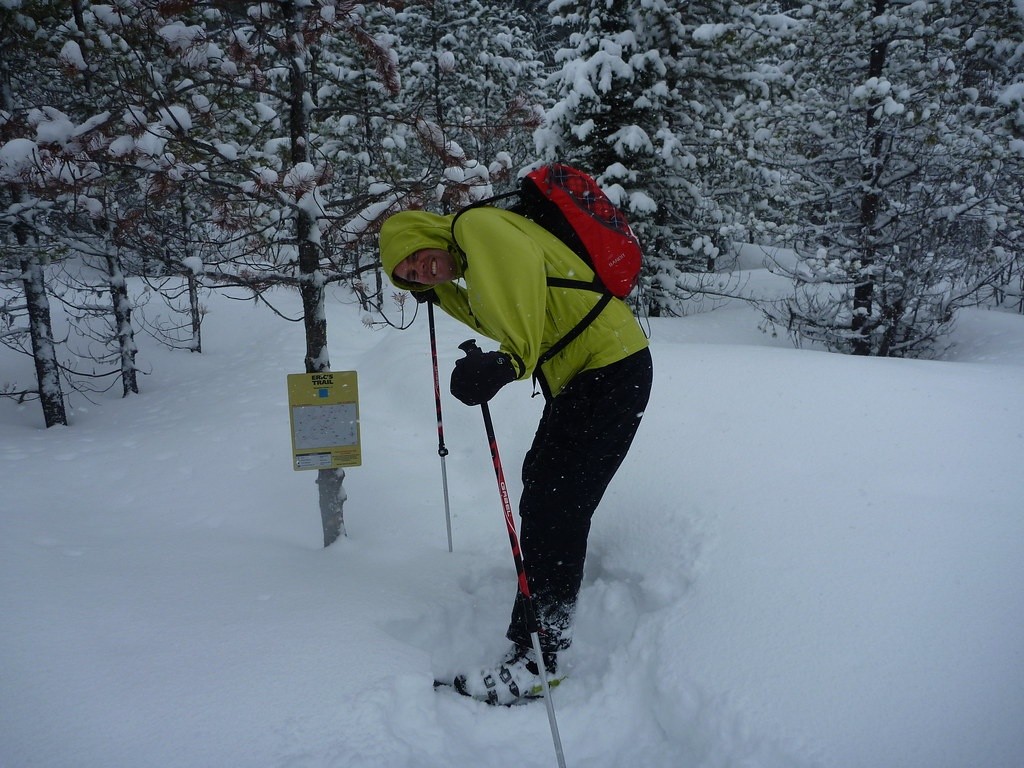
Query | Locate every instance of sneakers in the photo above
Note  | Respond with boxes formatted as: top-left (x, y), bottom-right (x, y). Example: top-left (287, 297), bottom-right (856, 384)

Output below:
top-left (455, 645), bottom-right (567, 706)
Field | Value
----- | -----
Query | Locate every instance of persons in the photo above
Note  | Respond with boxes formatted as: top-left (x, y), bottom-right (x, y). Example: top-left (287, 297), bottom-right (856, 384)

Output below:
top-left (378, 206), bottom-right (653, 708)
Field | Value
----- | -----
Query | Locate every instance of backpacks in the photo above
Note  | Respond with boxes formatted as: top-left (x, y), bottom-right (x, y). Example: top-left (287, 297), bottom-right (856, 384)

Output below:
top-left (449, 161), bottom-right (644, 364)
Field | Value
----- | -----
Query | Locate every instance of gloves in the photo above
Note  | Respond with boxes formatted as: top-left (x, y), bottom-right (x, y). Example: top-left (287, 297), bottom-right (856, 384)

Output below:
top-left (449, 351), bottom-right (515, 406)
top-left (410, 290), bottom-right (442, 303)
